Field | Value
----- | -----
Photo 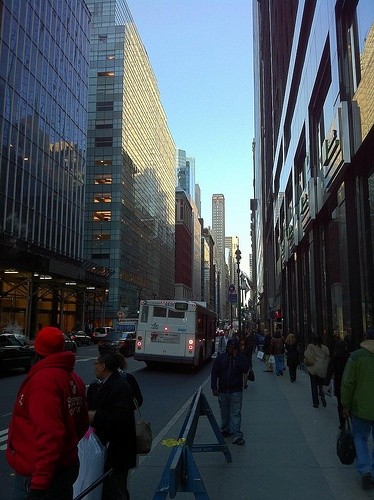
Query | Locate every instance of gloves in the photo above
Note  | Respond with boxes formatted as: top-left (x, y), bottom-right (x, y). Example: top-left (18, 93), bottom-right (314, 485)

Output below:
top-left (213, 390), bottom-right (219, 396)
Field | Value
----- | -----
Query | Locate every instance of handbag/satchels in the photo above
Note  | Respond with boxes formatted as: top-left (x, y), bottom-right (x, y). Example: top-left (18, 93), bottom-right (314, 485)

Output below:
top-left (268, 355), bottom-right (275, 364)
top-left (326, 378), bottom-right (334, 398)
top-left (304, 353), bottom-right (315, 366)
top-left (256, 351), bottom-right (264, 359)
top-left (247, 371), bottom-right (254, 381)
top-left (337, 415), bottom-right (356, 465)
top-left (134, 399), bottom-right (152, 456)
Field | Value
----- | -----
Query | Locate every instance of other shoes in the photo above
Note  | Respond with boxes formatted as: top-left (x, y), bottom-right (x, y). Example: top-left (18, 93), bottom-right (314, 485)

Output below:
top-left (313, 404), bottom-right (319, 408)
top-left (232, 438), bottom-right (245, 445)
top-left (322, 399), bottom-right (326, 407)
top-left (221, 431), bottom-right (231, 438)
top-left (276, 372), bottom-right (283, 376)
top-left (362, 475), bottom-right (374, 490)
top-left (243, 385), bottom-right (248, 389)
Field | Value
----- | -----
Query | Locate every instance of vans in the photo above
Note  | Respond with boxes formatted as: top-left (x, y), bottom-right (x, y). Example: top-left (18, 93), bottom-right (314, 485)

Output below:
top-left (115, 321), bottom-right (137, 332)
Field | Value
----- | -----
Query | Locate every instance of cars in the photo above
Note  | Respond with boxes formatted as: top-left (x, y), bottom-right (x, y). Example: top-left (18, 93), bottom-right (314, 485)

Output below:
top-left (101, 331), bottom-right (136, 358)
top-left (0, 333), bottom-right (39, 373)
top-left (62, 333), bottom-right (77, 354)
top-left (211, 319), bottom-right (231, 360)
top-left (66, 331), bottom-right (92, 347)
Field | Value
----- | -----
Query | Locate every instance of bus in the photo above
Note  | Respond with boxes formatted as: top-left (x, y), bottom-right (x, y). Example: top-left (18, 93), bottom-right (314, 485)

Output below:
top-left (135, 299), bottom-right (218, 372)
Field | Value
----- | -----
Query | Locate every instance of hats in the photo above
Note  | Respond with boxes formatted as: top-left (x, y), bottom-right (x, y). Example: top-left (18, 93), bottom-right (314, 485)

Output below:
top-left (34, 326), bottom-right (65, 357)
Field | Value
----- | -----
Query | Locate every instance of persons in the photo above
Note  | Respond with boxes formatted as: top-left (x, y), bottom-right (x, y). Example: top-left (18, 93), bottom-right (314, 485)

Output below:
top-left (214, 326), bottom-right (351, 431)
top-left (87, 349), bottom-right (143, 500)
top-left (211, 338), bottom-right (249, 446)
top-left (340, 332), bottom-right (374, 490)
top-left (6, 325), bottom-right (91, 500)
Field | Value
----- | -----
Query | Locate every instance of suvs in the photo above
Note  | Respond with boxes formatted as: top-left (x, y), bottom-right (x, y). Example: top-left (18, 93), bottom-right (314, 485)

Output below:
top-left (93, 327), bottom-right (113, 344)
